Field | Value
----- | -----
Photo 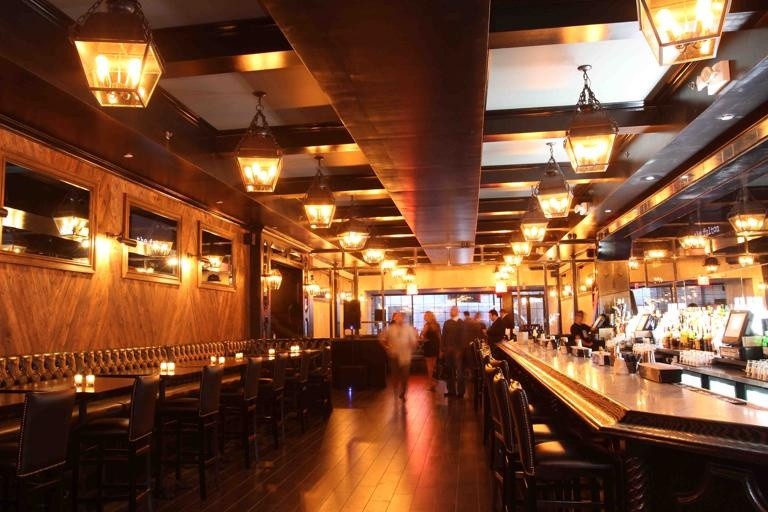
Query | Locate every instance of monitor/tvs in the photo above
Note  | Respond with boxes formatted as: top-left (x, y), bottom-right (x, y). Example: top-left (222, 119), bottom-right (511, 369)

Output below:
top-left (636, 314), bottom-right (652, 330)
top-left (597, 238), bottom-right (631, 261)
top-left (591, 315), bottom-right (605, 330)
top-left (722, 311), bottom-right (749, 345)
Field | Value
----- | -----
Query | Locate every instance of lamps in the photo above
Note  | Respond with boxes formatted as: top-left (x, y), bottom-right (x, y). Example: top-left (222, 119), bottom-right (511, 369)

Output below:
top-left (150, 225), bottom-right (175, 256)
top-left (549, 202), bottom-right (720, 302)
top-left (70, 2), bottom-right (165, 115)
top-left (634, 0), bottom-right (736, 64)
top-left (51, 187), bottom-right (91, 239)
top-left (533, 141), bottom-right (575, 218)
top-left (727, 172), bottom-right (767, 234)
top-left (230, 89), bottom-right (290, 194)
top-left (259, 263), bottom-right (286, 293)
top-left (493, 231), bottom-right (533, 286)
top-left (336, 190), bottom-right (416, 284)
top-left (520, 189), bottom-right (552, 242)
top-left (205, 237), bottom-right (227, 273)
top-left (300, 154), bottom-right (337, 232)
top-left (301, 272), bottom-right (320, 299)
top-left (562, 63), bottom-right (619, 174)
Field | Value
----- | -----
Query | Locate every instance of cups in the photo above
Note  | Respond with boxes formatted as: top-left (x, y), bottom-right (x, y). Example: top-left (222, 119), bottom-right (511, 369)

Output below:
top-left (210, 357), bottom-right (225, 365)
top-left (236, 353), bottom-right (243, 360)
top-left (290, 346), bottom-right (302, 352)
top-left (73, 376), bottom-right (96, 386)
top-left (160, 362), bottom-right (175, 371)
top-left (625, 360), bottom-right (639, 373)
top-left (583, 348), bottom-right (592, 359)
top-left (268, 349), bottom-right (276, 357)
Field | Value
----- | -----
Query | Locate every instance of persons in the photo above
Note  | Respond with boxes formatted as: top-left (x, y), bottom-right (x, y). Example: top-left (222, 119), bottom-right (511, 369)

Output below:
top-left (570, 311), bottom-right (598, 347)
top-left (378, 303), bottom-right (519, 403)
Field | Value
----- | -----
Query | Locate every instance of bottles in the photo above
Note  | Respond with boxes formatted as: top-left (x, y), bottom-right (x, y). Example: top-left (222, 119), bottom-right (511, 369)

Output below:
top-left (661, 305), bottom-right (732, 351)
top-left (744, 358), bottom-right (767, 380)
top-left (679, 350), bottom-right (716, 366)
top-left (611, 345), bottom-right (621, 365)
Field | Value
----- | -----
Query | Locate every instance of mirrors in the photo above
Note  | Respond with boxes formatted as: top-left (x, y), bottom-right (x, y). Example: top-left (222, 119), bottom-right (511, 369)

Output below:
top-left (619, 159), bottom-right (767, 317)
top-left (311, 265), bottom-right (332, 303)
top-left (119, 190), bottom-right (184, 288)
top-left (337, 274), bottom-right (355, 304)
top-left (0, 150), bottom-right (99, 275)
top-left (194, 219), bottom-right (238, 295)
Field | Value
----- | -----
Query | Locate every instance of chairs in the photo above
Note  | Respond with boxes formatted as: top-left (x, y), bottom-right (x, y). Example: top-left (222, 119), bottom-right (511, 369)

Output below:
top-left (469, 335), bottom-right (624, 510)
top-left (1, 343), bottom-right (335, 511)
